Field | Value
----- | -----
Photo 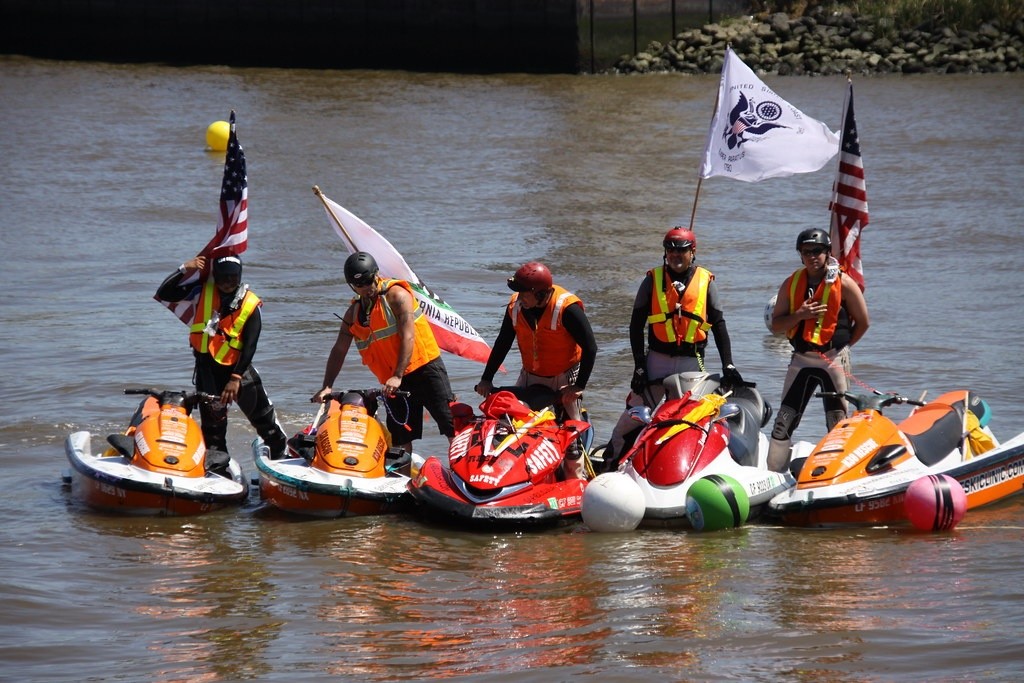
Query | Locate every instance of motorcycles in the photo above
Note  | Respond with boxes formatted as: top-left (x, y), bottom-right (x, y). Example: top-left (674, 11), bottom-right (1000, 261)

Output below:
top-left (61, 386), bottom-right (250, 515)
top-left (611, 371), bottom-right (818, 528)
top-left (250, 388), bottom-right (428, 519)
top-left (407, 384), bottom-right (603, 526)
top-left (768, 391), bottom-right (1024, 525)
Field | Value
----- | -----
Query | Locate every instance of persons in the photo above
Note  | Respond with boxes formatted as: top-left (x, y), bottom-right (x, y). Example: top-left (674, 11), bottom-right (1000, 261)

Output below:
top-left (475, 261), bottom-right (599, 480)
top-left (605, 226), bottom-right (733, 465)
top-left (156, 255), bottom-right (288, 479)
top-left (767, 228), bottom-right (870, 472)
top-left (314, 252), bottom-right (458, 452)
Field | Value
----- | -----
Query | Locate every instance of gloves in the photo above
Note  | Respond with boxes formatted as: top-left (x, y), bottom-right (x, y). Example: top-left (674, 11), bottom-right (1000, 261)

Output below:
top-left (631, 370), bottom-right (646, 395)
top-left (723, 367), bottom-right (744, 388)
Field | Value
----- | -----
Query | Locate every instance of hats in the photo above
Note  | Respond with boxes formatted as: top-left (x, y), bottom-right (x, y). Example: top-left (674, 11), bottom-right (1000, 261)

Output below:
top-left (212, 255), bottom-right (242, 277)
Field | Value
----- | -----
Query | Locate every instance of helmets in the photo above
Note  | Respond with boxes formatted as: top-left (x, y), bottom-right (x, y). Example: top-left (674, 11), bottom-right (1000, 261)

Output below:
top-left (507, 262), bottom-right (553, 294)
top-left (663, 226), bottom-right (696, 250)
top-left (344, 252), bottom-right (379, 283)
top-left (796, 228), bottom-right (832, 248)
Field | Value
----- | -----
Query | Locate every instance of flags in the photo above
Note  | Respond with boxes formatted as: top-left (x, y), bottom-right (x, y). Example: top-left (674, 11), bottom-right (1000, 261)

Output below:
top-left (318, 195), bottom-right (507, 374)
top-left (153, 112), bottom-right (250, 329)
top-left (826, 83), bottom-right (870, 293)
top-left (699, 48), bottom-right (840, 184)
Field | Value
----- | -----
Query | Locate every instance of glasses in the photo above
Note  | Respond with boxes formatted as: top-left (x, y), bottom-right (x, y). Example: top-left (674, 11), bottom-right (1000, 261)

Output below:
top-left (666, 246), bottom-right (689, 254)
top-left (355, 274), bottom-right (374, 287)
top-left (803, 247), bottom-right (825, 256)
